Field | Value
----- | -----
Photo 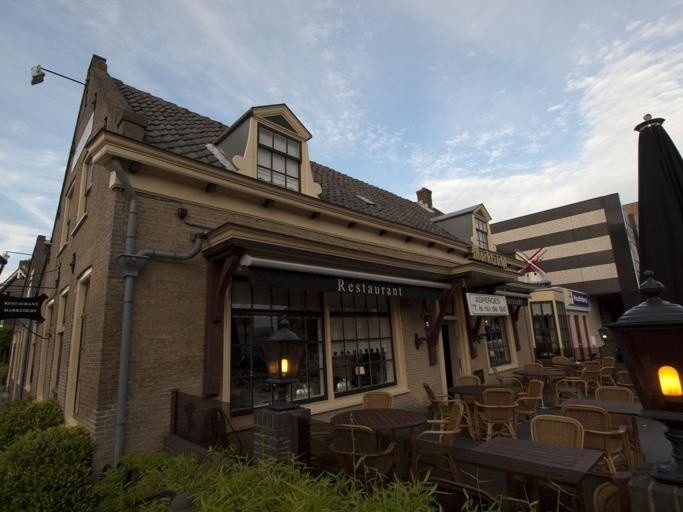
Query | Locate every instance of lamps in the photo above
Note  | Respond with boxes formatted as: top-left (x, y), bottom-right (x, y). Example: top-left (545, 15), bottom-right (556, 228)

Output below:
top-left (476, 319), bottom-right (488, 343)
top-left (415, 319), bottom-right (432, 349)
top-left (30, 64), bottom-right (87, 86)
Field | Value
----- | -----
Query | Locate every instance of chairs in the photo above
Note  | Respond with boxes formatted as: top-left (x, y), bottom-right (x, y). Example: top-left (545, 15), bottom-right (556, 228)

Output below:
top-left (329, 423), bottom-right (400, 491)
top-left (362, 390), bottom-right (394, 410)
top-left (513, 415), bottom-right (586, 511)
top-left (421, 355), bottom-right (646, 475)
top-left (426, 477), bottom-right (502, 512)
top-left (405, 401), bottom-right (464, 485)
top-left (331, 410), bottom-right (427, 482)
top-left (593, 481), bottom-right (623, 511)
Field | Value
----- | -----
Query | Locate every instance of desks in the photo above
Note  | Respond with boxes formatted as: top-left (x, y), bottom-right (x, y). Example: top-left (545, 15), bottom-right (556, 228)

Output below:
top-left (469, 435), bottom-right (607, 511)
